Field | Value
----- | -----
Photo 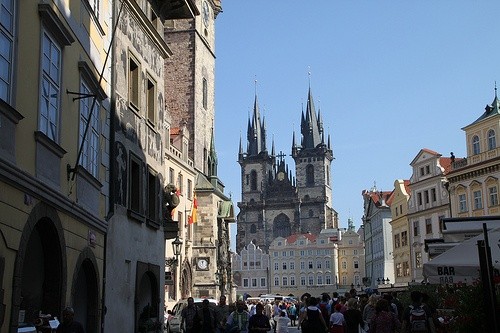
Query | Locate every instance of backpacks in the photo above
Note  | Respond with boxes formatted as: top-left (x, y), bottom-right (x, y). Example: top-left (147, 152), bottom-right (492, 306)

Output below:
top-left (301, 307), bottom-right (322, 333)
top-left (408, 305), bottom-right (428, 333)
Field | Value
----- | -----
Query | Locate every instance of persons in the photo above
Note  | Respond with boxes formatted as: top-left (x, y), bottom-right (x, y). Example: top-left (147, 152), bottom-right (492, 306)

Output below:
top-left (262, 301), bottom-right (302, 330)
top-left (249, 303), bottom-right (271, 333)
top-left (421, 293), bottom-right (441, 333)
top-left (56, 306), bottom-right (83, 333)
top-left (400, 291), bottom-right (435, 333)
top-left (228, 300), bottom-right (251, 333)
top-left (297, 297), bottom-right (328, 333)
top-left (363, 296), bottom-right (377, 333)
top-left (274, 312), bottom-right (291, 333)
top-left (369, 299), bottom-right (400, 333)
top-left (181, 297), bottom-right (199, 333)
top-left (197, 300), bottom-right (217, 333)
top-left (299, 296), bottom-right (308, 316)
top-left (319, 289), bottom-right (368, 333)
top-left (223, 300), bottom-right (258, 324)
top-left (383, 292), bottom-right (404, 333)
top-left (214, 296), bottom-right (228, 333)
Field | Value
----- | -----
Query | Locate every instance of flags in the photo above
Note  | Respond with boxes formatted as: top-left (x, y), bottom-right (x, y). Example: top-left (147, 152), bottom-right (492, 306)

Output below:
top-left (188, 191), bottom-right (197, 224)
top-left (166, 185), bottom-right (179, 218)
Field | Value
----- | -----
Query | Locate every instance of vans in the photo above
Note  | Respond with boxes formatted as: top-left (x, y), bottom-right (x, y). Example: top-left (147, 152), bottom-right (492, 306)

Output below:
top-left (260, 295), bottom-right (295, 304)
top-left (166, 298), bottom-right (219, 333)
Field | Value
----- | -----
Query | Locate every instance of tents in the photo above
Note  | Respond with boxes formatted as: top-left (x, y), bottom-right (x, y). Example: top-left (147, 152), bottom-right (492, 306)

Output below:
top-left (423, 225), bottom-right (500, 286)
